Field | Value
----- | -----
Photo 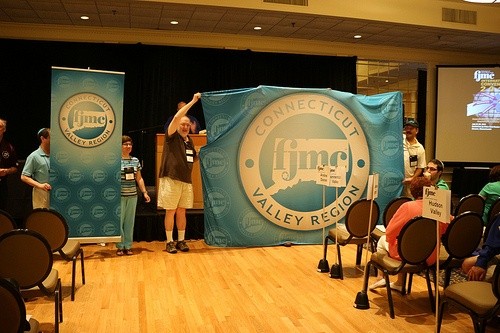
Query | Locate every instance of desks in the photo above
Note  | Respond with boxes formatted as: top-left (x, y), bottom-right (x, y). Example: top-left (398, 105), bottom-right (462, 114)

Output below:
top-left (155, 134), bottom-right (207, 210)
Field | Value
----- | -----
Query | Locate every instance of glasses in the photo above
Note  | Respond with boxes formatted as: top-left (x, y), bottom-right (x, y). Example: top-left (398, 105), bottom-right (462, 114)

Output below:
top-left (425, 166), bottom-right (437, 171)
top-left (121, 142), bottom-right (134, 147)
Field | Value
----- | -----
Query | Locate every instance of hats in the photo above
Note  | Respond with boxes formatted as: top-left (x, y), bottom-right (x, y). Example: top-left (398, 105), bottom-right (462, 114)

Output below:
top-left (405, 120), bottom-right (419, 128)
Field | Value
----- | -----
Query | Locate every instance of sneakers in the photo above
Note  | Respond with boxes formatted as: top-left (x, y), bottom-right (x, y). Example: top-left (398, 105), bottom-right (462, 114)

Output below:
top-left (176, 241), bottom-right (190, 251)
top-left (166, 241), bottom-right (177, 253)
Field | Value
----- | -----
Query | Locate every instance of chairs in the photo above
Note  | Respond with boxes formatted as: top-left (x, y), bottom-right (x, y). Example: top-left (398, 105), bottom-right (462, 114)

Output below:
top-left (324, 199), bottom-right (380, 280)
top-left (364, 218), bottom-right (437, 319)
top-left (25, 209), bottom-right (85, 301)
top-left (437, 262), bottom-right (500, 333)
top-left (455, 194), bottom-right (485, 217)
top-left (432, 210), bottom-right (483, 289)
top-left (0, 228), bottom-right (63, 333)
top-left (371, 196), bottom-right (414, 249)
top-left (488, 198), bottom-right (500, 222)
top-left (0, 277), bottom-right (39, 333)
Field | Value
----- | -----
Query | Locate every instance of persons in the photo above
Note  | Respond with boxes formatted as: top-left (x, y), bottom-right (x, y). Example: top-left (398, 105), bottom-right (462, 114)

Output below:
top-left (155, 92), bottom-right (204, 252)
top-left (161, 101), bottom-right (201, 134)
top-left (366, 178), bottom-right (447, 294)
top-left (0, 118), bottom-right (20, 219)
top-left (116, 135), bottom-right (151, 256)
top-left (398, 120), bottom-right (426, 202)
top-left (20, 127), bottom-right (52, 212)
top-left (458, 161), bottom-right (500, 329)
top-left (425, 159), bottom-right (451, 192)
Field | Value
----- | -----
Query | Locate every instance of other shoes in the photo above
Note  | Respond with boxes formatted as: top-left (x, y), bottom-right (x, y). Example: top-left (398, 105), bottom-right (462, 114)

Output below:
top-left (368, 278), bottom-right (386, 290)
top-left (390, 282), bottom-right (402, 292)
top-left (116, 248), bottom-right (133, 256)
